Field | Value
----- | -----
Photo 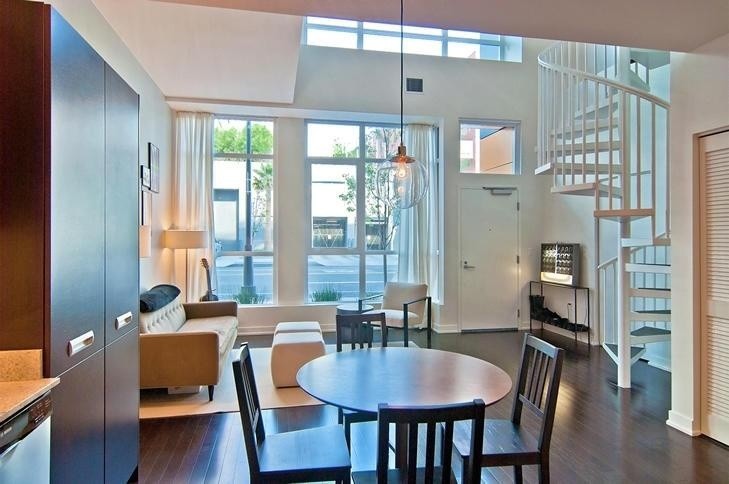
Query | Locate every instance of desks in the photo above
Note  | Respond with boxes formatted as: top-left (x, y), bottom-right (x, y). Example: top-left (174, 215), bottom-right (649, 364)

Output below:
top-left (294, 344), bottom-right (515, 483)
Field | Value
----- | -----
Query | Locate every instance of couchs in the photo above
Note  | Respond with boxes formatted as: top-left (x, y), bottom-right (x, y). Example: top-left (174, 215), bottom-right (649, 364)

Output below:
top-left (136, 278), bottom-right (240, 402)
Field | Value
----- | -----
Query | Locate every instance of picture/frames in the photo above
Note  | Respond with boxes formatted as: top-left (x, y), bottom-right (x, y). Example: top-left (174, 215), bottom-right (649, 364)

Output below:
top-left (141, 163), bottom-right (151, 189)
top-left (147, 141), bottom-right (161, 195)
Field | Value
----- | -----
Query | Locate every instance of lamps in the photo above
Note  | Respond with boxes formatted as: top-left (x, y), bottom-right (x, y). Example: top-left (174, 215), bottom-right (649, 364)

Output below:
top-left (139, 223), bottom-right (152, 262)
top-left (372, 0), bottom-right (428, 209)
top-left (165, 226), bottom-right (210, 303)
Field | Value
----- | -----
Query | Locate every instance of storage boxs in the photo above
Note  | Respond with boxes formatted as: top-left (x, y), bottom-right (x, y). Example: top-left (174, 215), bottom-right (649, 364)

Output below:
top-left (164, 382), bottom-right (205, 398)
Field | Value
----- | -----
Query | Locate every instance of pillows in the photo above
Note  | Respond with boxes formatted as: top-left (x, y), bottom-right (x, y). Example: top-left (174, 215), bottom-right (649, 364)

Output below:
top-left (139, 283), bottom-right (184, 312)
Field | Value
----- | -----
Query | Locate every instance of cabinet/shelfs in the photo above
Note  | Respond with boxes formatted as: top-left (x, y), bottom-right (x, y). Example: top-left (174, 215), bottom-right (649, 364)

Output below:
top-left (525, 278), bottom-right (591, 359)
top-left (0, 0), bottom-right (144, 483)
top-left (0, 414), bottom-right (53, 484)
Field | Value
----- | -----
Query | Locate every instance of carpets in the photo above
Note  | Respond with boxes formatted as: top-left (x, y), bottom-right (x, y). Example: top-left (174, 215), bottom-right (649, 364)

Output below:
top-left (138, 337), bottom-right (425, 426)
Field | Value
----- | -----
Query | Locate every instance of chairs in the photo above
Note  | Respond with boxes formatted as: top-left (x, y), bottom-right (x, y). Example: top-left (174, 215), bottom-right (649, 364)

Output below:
top-left (230, 338), bottom-right (356, 483)
top-left (436, 325), bottom-right (566, 484)
top-left (349, 392), bottom-right (489, 484)
top-left (331, 311), bottom-right (392, 465)
top-left (354, 275), bottom-right (436, 350)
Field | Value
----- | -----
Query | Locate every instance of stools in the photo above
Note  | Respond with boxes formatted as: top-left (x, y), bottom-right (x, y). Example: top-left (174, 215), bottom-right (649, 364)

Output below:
top-left (335, 302), bottom-right (374, 344)
top-left (268, 331), bottom-right (327, 388)
top-left (272, 319), bottom-right (322, 334)
